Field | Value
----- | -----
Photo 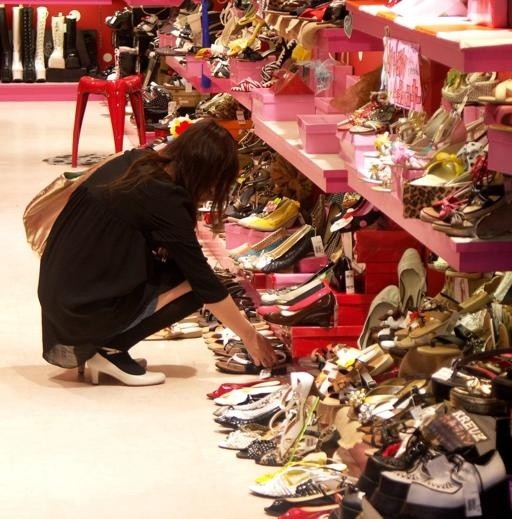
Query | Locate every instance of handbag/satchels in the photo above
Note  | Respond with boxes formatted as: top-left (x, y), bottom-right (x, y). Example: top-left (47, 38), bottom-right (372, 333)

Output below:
top-left (23, 147), bottom-right (139, 258)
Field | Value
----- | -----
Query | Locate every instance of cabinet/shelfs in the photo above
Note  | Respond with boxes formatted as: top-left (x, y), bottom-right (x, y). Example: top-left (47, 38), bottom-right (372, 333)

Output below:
top-left (0, 0), bottom-right (207, 102)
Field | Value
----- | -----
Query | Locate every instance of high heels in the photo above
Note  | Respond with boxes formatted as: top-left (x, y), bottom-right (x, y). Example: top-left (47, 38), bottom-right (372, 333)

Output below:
top-left (73, 345), bottom-right (167, 386)
top-left (98, 1), bottom-right (353, 131)
top-left (329, 64), bottom-right (512, 241)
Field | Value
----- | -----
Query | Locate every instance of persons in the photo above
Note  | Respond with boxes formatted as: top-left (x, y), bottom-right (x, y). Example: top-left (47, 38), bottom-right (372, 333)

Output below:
top-left (35, 118), bottom-right (277, 388)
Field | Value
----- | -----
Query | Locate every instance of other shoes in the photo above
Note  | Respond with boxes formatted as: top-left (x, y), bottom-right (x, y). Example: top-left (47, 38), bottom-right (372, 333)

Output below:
top-left (203, 132), bottom-right (367, 373)
top-left (206, 246), bottom-right (511, 519)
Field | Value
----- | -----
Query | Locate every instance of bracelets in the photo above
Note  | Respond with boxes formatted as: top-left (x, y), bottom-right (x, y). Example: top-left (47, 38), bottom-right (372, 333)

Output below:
top-left (240, 323), bottom-right (255, 345)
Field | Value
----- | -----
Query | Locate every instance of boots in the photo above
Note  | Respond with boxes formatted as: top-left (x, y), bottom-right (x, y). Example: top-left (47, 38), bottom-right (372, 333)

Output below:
top-left (78, 29), bottom-right (100, 78)
top-left (12, 5), bottom-right (50, 83)
top-left (47, 15), bottom-right (80, 70)
top-left (0, 3), bottom-right (11, 83)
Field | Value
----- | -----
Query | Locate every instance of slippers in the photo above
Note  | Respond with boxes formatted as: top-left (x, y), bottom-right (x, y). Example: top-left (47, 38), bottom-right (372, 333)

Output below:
top-left (143, 314), bottom-right (202, 343)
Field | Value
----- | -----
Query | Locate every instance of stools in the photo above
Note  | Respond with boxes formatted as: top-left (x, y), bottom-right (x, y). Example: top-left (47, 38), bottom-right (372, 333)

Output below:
top-left (71, 74), bottom-right (145, 168)
